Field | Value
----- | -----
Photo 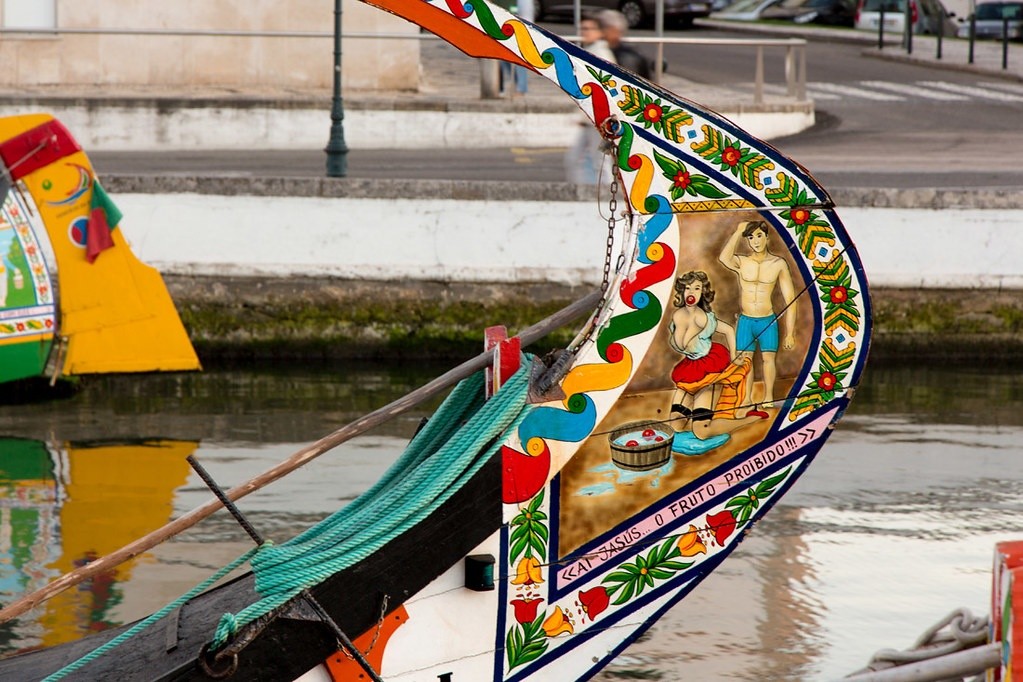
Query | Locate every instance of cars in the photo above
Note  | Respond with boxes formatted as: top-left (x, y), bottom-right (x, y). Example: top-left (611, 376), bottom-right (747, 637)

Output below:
top-left (710, 0), bottom-right (775, 22)
top-left (760, 1), bottom-right (854, 28)
top-left (957, 0), bottom-right (1023, 41)
top-left (532, 0), bottom-right (710, 29)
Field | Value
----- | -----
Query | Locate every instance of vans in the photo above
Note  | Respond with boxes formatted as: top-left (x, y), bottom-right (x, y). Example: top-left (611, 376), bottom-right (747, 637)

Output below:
top-left (854, 0), bottom-right (960, 36)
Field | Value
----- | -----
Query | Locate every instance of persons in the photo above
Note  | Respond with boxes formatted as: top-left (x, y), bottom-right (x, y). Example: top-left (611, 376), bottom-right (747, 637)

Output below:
top-left (568, 9), bottom-right (652, 186)
top-left (498, 0), bottom-right (536, 100)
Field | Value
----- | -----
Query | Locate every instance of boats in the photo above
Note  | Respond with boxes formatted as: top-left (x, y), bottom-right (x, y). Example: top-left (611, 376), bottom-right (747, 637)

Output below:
top-left (1, 113), bottom-right (202, 386)
top-left (1, 0), bottom-right (1023, 682)
